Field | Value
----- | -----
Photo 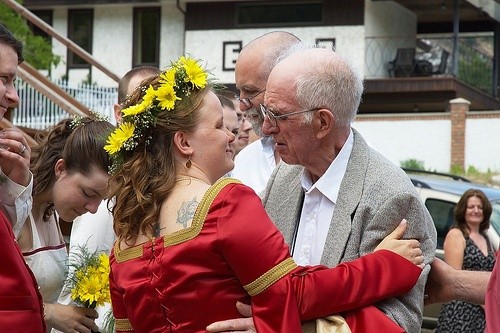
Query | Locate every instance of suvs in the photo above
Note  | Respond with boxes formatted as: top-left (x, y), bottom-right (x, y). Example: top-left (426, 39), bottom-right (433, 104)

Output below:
top-left (400, 168), bottom-right (499, 328)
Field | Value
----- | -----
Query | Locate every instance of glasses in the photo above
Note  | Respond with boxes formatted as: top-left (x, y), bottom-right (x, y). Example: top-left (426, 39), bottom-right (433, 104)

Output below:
top-left (259, 103), bottom-right (323, 126)
top-left (234, 89), bottom-right (266, 107)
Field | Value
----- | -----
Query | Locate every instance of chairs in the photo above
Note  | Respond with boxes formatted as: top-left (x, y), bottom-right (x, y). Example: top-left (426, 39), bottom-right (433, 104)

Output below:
top-left (389, 48), bottom-right (450, 78)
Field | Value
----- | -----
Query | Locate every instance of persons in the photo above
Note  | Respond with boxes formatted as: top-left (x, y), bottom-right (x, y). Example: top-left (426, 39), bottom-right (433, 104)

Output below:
top-left (205, 39), bottom-right (438, 333)
top-left (103, 54), bottom-right (425, 333)
top-left (224, 31), bottom-right (307, 204)
top-left (216, 89), bottom-right (254, 159)
top-left (435, 189), bottom-right (495, 333)
top-left (216, 95), bottom-right (240, 160)
top-left (0, 23), bottom-right (49, 333)
top-left (423, 236), bottom-right (500, 333)
top-left (15, 113), bottom-right (117, 333)
top-left (51, 66), bottom-right (163, 333)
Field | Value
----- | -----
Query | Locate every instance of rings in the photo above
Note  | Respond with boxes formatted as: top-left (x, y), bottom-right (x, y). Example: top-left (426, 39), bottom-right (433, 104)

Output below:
top-left (19, 145), bottom-right (28, 156)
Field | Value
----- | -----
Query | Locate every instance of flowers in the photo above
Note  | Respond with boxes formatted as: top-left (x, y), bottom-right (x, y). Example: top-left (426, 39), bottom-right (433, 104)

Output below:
top-left (57, 234), bottom-right (112, 308)
top-left (103, 56), bottom-right (208, 155)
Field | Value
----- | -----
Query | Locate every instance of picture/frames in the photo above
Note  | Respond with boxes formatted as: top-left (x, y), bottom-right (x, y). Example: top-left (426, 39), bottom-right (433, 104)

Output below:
top-left (315, 38), bottom-right (336, 52)
top-left (223, 41), bottom-right (242, 71)
